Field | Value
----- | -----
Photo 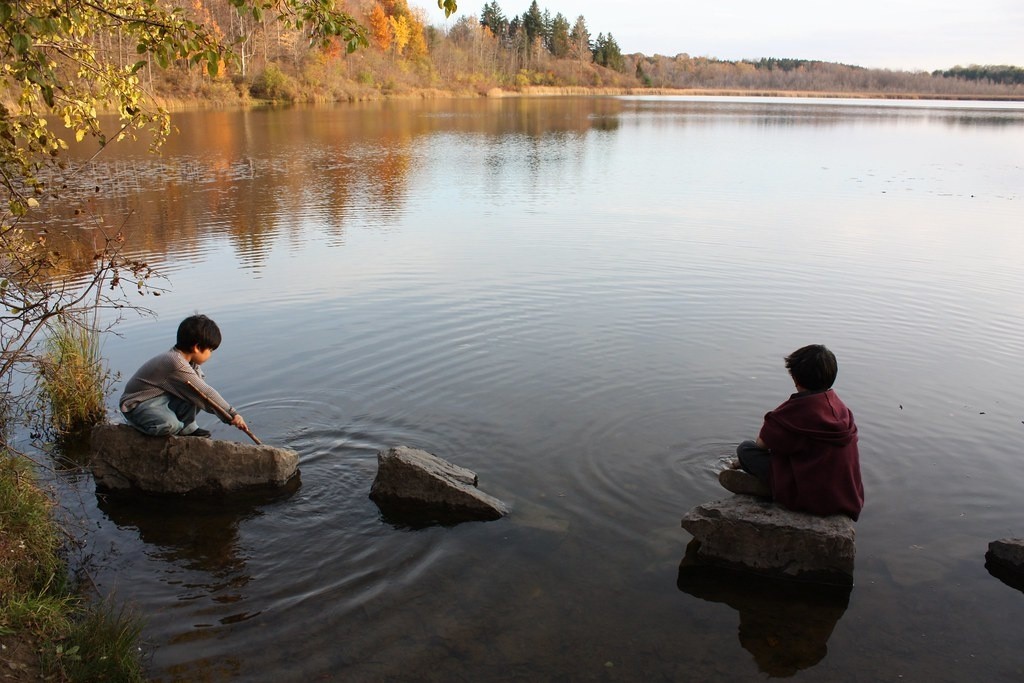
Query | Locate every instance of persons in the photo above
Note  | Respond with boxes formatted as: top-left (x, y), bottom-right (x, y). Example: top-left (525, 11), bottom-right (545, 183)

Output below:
top-left (719, 344), bottom-right (865, 522)
top-left (120, 314), bottom-right (248, 438)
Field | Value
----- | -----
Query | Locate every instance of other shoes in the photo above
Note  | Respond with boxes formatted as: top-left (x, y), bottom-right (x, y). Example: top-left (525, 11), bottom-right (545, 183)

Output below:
top-left (181, 428), bottom-right (213, 438)
top-left (719, 469), bottom-right (773, 499)
